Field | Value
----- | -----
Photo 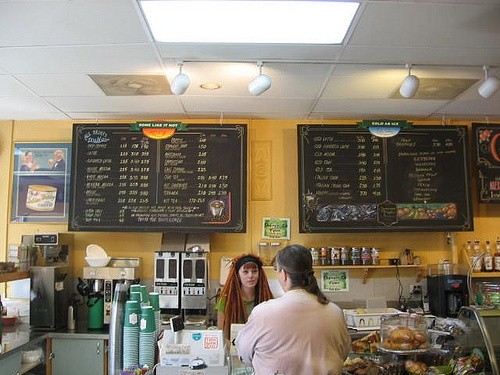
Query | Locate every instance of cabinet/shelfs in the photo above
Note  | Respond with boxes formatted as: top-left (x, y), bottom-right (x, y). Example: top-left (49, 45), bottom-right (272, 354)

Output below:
top-left (46, 339), bottom-right (107, 375)
top-left (460, 246), bottom-right (500, 317)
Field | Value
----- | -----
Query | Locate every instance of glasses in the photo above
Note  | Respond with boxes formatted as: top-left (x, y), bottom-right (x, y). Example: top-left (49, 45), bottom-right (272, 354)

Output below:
top-left (274, 266), bottom-right (280, 271)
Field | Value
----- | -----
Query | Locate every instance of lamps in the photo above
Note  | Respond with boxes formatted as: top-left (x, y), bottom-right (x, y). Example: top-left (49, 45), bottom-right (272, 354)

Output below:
top-left (477, 66), bottom-right (500, 100)
top-left (171, 61), bottom-right (191, 95)
top-left (399, 64), bottom-right (420, 99)
top-left (248, 60), bottom-right (273, 95)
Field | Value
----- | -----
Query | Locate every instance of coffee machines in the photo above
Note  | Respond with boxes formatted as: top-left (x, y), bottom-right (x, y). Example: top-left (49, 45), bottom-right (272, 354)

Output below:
top-left (426, 261), bottom-right (469, 320)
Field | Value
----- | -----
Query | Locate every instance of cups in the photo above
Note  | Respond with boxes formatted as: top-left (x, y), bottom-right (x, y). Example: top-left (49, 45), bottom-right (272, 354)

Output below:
top-left (107, 284), bottom-right (161, 375)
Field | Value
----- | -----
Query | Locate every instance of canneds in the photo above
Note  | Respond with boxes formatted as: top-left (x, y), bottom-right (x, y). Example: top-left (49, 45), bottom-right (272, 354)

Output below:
top-left (310, 246), bottom-right (381, 265)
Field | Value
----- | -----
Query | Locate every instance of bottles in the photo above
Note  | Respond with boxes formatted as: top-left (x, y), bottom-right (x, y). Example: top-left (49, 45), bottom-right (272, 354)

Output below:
top-left (87, 292), bottom-right (103, 329)
top-left (67, 304), bottom-right (77, 330)
top-left (311, 246), bottom-right (380, 266)
top-left (462, 240), bottom-right (500, 272)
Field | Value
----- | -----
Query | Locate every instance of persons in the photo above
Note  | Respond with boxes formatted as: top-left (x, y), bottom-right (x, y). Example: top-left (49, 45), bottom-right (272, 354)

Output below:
top-left (232, 245), bottom-right (351, 375)
top-left (214, 252), bottom-right (274, 347)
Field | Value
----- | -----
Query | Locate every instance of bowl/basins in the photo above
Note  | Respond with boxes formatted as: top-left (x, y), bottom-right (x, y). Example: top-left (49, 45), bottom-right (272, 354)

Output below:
top-left (84, 244), bottom-right (113, 266)
top-left (20, 347), bottom-right (43, 363)
top-left (1, 316), bottom-right (17, 327)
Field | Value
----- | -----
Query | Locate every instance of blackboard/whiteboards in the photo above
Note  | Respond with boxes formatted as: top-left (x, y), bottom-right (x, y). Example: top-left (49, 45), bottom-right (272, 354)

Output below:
top-left (68, 123), bottom-right (247, 233)
top-left (472, 122), bottom-right (500, 203)
top-left (297, 123), bottom-right (474, 233)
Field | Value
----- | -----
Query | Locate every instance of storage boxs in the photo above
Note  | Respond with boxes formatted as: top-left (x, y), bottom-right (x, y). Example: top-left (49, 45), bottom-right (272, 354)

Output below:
top-left (344, 307), bottom-right (404, 329)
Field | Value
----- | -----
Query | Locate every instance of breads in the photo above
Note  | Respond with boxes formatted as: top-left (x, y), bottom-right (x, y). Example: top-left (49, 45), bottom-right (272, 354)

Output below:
top-left (342, 326), bottom-right (430, 375)
